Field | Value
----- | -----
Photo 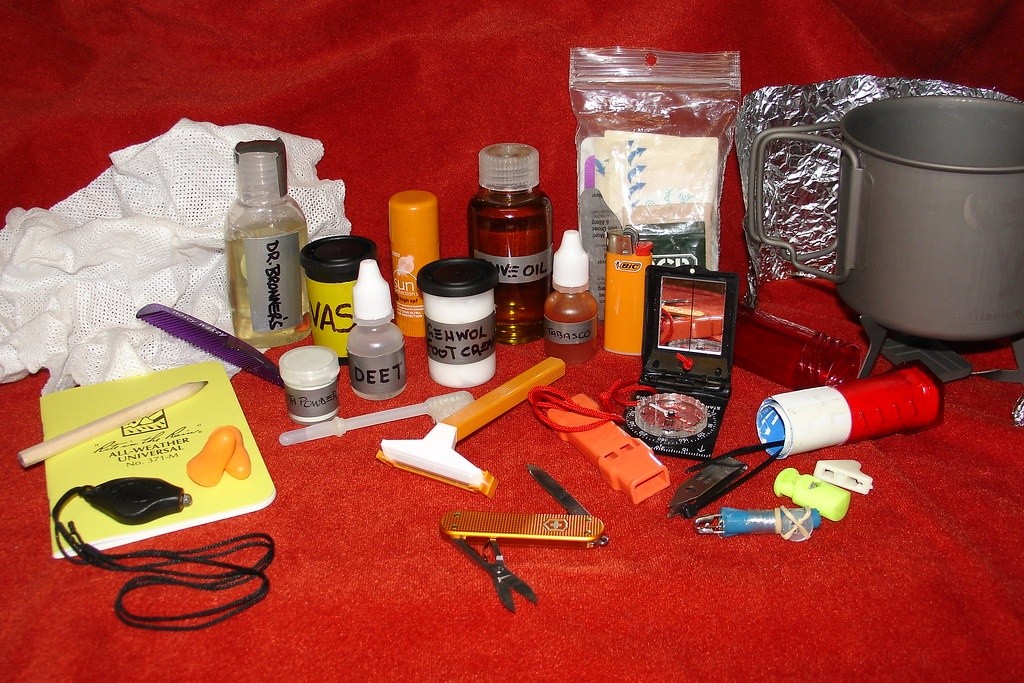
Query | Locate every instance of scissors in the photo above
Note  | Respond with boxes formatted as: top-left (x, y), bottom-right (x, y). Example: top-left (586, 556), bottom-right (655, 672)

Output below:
top-left (452, 537), bottom-right (538, 615)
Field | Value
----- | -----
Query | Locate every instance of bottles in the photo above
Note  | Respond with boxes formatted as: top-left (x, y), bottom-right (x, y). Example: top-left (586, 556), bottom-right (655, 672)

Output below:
top-left (346, 258), bottom-right (408, 400)
top-left (388, 189), bottom-right (440, 338)
top-left (544, 230), bottom-right (598, 344)
top-left (473, 141), bottom-right (554, 340)
top-left (224, 137), bottom-right (313, 348)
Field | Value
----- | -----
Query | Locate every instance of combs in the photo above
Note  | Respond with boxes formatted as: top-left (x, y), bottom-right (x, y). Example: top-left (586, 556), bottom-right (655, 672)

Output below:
top-left (137, 303), bottom-right (285, 389)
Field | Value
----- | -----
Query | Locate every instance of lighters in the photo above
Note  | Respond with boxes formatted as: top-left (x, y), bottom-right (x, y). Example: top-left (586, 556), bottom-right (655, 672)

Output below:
top-left (603, 226), bottom-right (653, 356)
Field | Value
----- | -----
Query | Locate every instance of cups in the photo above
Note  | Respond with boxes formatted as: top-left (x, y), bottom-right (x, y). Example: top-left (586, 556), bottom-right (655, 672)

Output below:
top-left (417, 257), bottom-right (495, 386)
top-left (300, 236), bottom-right (378, 364)
top-left (748, 96), bottom-right (1024, 342)
top-left (279, 344), bottom-right (342, 426)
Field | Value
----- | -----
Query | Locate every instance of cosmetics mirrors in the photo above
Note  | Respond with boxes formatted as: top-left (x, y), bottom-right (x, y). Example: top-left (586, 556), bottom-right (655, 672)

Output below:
top-left (658, 276), bottom-right (727, 355)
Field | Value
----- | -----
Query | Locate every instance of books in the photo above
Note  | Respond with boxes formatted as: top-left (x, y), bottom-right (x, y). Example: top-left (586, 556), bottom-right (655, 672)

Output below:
top-left (40, 361), bottom-right (275, 559)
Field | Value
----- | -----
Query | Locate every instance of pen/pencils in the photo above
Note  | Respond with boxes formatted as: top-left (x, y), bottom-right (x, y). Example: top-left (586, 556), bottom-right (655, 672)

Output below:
top-left (17, 381), bottom-right (211, 468)
